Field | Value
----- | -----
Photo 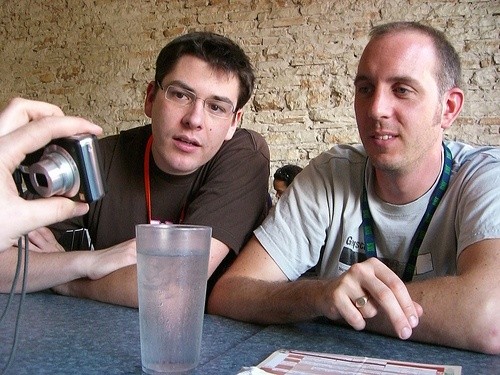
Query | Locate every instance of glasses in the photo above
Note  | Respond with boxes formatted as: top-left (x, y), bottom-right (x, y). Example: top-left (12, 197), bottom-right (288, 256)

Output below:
top-left (156, 79), bottom-right (243, 119)
top-left (276, 168), bottom-right (292, 182)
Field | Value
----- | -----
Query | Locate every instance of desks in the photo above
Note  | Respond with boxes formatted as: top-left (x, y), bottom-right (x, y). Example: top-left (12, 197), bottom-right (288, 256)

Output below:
top-left (0, 291), bottom-right (500, 375)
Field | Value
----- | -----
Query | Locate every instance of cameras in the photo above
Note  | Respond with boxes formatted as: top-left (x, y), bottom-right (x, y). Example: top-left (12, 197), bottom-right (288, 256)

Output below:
top-left (16, 132), bottom-right (108, 203)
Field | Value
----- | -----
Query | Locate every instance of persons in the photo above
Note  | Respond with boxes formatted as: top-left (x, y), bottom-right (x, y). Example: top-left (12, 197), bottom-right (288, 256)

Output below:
top-left (0, 32), bottom-right (271, 308)
top-left (207, 22), bottom-right (500, 354)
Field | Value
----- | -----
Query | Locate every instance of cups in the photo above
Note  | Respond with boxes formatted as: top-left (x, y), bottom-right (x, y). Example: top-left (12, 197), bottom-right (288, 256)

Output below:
top-left (134, 224), bottom-right (213, 375)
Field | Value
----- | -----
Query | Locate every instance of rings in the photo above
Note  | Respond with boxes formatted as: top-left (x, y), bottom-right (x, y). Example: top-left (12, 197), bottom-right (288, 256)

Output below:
top-left (356, 294), bottom-right (371, 307)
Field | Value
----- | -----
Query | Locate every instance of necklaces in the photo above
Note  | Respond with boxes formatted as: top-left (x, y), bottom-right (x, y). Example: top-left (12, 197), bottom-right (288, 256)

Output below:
top-left (144, 134), bottom-right (185, 224)
top-left (362, 139), bottom-right (452, 284)
